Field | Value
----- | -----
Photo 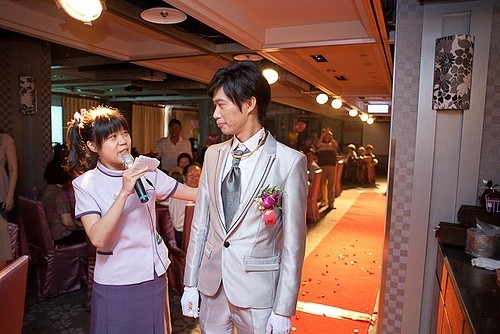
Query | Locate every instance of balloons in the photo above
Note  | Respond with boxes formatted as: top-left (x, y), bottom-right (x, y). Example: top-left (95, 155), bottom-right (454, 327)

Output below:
top-left (295, 121), bottom-right (307, 132)
top-left (287, 131), bottom-right (300, 146)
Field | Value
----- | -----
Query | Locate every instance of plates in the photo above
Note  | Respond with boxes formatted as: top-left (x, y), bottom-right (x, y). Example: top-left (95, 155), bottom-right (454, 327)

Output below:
top-left (468, 228), bottom-right (500, 259)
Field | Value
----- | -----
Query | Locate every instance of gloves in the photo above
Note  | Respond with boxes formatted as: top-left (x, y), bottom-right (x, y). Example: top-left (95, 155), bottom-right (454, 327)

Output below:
top-left (266, 311), bottom-right (292, 334)
top-left (180, 287), bottom-right (201, 319)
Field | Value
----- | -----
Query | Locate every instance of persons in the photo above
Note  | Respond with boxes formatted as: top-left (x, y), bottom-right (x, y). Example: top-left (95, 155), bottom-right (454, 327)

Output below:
top-left (131, 147), bottom-right (140, 162)
top-left (179, 60), bottom-right (308, 334)
top-left (62, 104), bottom-right (198, 334)
top-left (155, 118), bottom-right (194, 175)
top-left (168, 152), bottom-right (194, 184)
top-left (188, 137), bottom-right (210, 167)
top-left (38, 157), bottom-right (97, 258)
top-left (295, 126), bottom-right (379, 210)
top-left (0, 133), bottom-right (24, 257)
top-left (168, 161), bottom-right (203, 249)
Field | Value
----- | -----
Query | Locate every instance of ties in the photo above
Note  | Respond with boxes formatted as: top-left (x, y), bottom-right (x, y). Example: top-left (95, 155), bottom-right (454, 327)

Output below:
top-left (224, 146), bottom-right (251, 232)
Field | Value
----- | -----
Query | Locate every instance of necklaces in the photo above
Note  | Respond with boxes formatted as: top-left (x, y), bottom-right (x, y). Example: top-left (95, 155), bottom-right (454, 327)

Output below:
top-left (231, 129), bottom-right (266, 159)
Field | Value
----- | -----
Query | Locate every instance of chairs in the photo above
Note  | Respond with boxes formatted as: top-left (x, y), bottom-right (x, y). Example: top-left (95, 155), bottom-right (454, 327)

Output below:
top-left (166, 204), bottom-right (195, 300)
top-left (0, 254), bottom-right (29, 334)
top-left (335, 159), bottom-right (344, 198)
top-left (16, 194), bottom-right (87, 303)
top-left (345, 156), bottom-right (376, 186)
top-left (306, 168), bottom-right (323, 223)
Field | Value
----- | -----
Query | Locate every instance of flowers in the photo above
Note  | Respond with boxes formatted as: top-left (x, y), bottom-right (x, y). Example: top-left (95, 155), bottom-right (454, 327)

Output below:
top-left (253, 184), bottom-right (289, 227)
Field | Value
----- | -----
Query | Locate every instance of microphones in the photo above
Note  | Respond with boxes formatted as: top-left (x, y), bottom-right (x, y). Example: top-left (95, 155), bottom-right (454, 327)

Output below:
top-left (121, 153), bottom-right (149, 203)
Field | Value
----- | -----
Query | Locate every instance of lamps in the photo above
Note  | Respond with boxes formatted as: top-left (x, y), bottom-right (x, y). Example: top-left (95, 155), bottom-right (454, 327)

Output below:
top-left (348, 108), bottom-right (374, 125)
top-left (140, 2), bottom-right (187, 26)
top-left (315, 93), bottom-right (329, 105)
top-left (261, 67), bottom-right (279, 85)
top-left (53, 0), bottom-right (108, 27)
top-left (331, 98), bottom-right (342, 109)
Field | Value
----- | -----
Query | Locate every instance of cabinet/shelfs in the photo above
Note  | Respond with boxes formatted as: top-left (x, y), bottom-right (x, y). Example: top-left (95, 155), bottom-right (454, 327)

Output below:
top-left (427, 242), bottom-right (500, 334)
